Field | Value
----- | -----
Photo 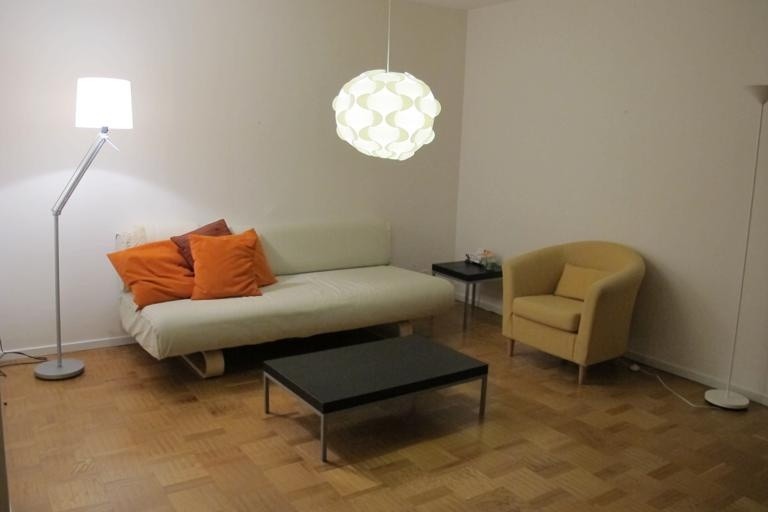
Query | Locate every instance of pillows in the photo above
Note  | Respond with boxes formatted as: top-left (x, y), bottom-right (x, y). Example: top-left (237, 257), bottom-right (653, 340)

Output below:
top-left (237, 227), bottom-right (278, 288)
top-left (188, 231), bottom-right (262, 300)
top-left (105, 240), bottom-right (194, 312)
top-left (554, 263), bottom-right (610, 300)
top-left (170, 219), bottom-right (231, 272)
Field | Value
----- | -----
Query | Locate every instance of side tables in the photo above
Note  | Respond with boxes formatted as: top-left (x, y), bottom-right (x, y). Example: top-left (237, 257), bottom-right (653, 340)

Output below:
top-left (432, 260), bottom-right (502, 331)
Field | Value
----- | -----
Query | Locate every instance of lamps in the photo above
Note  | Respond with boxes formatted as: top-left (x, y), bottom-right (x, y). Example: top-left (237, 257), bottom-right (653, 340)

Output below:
top-left (703, 84), bottom-right (768, 411)
top-left (34, 77), bottom-right (134, 381)
top-left (332, 0), bottom-right (441, 161)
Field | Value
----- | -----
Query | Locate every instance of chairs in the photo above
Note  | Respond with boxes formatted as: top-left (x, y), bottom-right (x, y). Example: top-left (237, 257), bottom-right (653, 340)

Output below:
top-left (502, 240), bottom-right (646, 385)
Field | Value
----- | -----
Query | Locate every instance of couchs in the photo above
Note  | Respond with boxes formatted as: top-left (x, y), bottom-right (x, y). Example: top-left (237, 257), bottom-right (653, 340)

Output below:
top-left (114, 216), bottom-right (455, 378)
